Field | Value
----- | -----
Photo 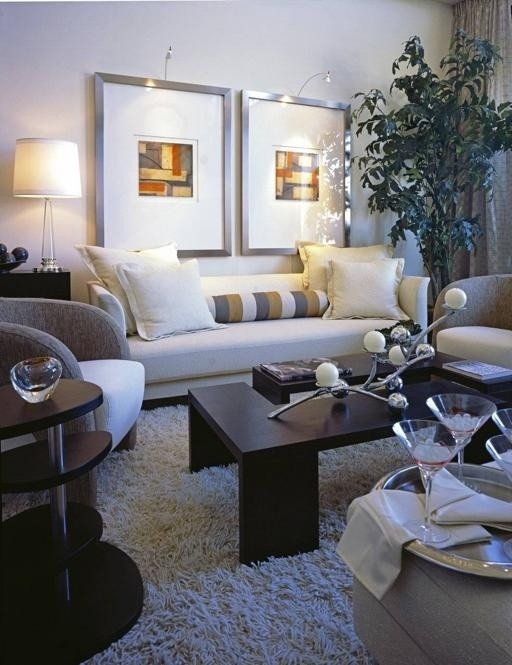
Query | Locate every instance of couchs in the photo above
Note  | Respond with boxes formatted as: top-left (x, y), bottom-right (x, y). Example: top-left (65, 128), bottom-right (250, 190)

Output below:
top-left (86, 272), bottom-right (435, 400)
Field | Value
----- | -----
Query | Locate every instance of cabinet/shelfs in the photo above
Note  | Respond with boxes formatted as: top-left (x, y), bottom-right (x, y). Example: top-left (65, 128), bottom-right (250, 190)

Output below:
top-left (0, 269), bottom-right (71, 301)
top-left (0, 377), bottom-right (144, 665)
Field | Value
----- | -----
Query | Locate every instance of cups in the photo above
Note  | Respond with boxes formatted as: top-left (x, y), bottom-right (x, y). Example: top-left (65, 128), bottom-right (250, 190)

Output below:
top-left (490, 406), bottom-right (512, 440)
top-left (11, 355), bottom-right (64, 404)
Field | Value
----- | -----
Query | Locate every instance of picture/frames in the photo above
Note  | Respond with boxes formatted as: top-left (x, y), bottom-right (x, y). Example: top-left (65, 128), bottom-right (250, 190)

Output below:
top-left (240, 87), bottom-right (352, 256)
top-left (94, 72), bottom-right (234, 258)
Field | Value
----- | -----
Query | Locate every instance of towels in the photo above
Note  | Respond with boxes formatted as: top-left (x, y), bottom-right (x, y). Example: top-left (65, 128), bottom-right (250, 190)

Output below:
top-left (335, 468), bottom-right (512, 602)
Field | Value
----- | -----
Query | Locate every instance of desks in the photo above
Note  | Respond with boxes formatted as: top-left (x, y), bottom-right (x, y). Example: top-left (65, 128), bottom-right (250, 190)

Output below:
top-left (352, 545), bottom-right (512, 665)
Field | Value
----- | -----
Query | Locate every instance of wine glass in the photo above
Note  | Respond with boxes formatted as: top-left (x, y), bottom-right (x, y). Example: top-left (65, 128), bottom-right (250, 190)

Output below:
top-left (484, 432), bottom-right (511, 563)
top-left (392, 416), bottom-right (471, 542)
top-left (426, 395), bottom-right (498, 495)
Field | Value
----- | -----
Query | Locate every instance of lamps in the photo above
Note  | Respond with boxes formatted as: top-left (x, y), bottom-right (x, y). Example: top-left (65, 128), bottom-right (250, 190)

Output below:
top-left (164, 45), bottom-right (173, 80)
top-left (297, 71), bottom-right (332, 96)
top-left (12, 138), bottom-right (82, 272)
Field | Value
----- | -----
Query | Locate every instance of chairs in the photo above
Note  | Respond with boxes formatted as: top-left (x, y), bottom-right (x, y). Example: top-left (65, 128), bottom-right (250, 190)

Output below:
top-left (0, 297), bottom-right (146, 507)
top-left (432, 272), bottom-right (512, 370)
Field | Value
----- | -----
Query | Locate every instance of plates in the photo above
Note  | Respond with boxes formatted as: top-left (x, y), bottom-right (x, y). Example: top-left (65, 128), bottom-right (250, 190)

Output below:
top-left (1, 259), bottom-right (26, 274)
top-left (371, 462), bottom-right (511, 581)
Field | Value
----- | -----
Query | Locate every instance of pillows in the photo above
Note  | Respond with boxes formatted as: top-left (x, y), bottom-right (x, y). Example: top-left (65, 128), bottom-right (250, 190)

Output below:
top-left (111, 258), bottom-right (228, 341)
top-left (75, 242), bottom-right (181, 335)
top-left (207, 289), bottom-right (328, 322)
top-left (321, 255), bottom-right (411, 322)
top-left (296, 239), bottom-right (396, 291)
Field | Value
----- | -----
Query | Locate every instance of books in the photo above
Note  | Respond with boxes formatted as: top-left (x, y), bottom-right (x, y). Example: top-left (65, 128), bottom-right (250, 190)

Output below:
top-left (442, 359), bottom-right (512, 385)
top-left (260, 356), bottom-right (353, 382)
